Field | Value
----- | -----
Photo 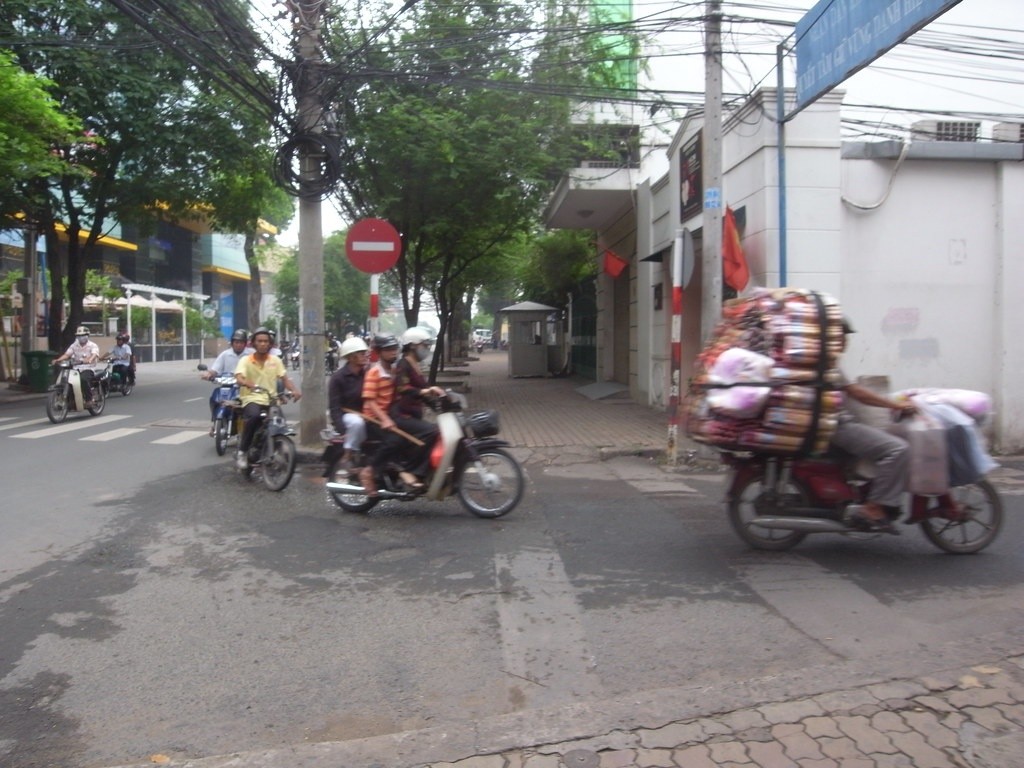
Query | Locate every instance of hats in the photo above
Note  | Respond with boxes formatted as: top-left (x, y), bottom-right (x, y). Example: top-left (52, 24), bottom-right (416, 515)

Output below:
top-left (841, 319), bottom-right (854, 334)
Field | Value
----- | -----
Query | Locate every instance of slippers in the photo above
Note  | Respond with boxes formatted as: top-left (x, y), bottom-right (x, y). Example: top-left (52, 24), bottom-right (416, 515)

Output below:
top-left (398, 472), bottom-right (424, 488)
top-left (856, 507), bottom-right (899, 536)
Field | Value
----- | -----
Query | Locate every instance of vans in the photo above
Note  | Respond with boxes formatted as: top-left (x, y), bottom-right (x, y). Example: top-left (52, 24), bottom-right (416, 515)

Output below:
top-left (473, 329), bottom-right (493, 344)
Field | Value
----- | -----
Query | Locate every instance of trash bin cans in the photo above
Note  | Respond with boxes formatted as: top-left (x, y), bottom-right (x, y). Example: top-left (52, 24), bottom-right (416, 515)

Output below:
top-left (20, 350), bottom-right (60, 392)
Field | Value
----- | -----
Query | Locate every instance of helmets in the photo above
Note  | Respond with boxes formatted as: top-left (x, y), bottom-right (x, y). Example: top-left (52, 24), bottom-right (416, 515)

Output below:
top-left (116, 335), bottom-right (123, 340)
top-left (328, 332), bottom-right (333, 338)
top-left (246, 333), bottom-right (253, 341)
top-left (339, 337), bottom-right (369, 359)
top-left (122, 334), bottom-right (129, 340)
top-left (403, 326), bottom-right (437, 346)
top-left (372, 333), bottom-right (398, 348)
top-left (251, 328), bottom-right (276, 348)
top-left (232, 328), bottom-right (245, 341)
top-left (74, 327), bottom-right (90, 337)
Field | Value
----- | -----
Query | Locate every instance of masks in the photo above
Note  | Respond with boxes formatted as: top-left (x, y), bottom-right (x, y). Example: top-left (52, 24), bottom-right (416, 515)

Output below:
top-left (415, 345), bottom-right (430, 360)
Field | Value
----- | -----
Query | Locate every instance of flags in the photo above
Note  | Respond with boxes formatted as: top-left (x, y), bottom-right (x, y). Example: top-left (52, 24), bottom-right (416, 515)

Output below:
top-left (604, 253), bottom-right (626, 276)
top-left (722, 208), bottom-right (749, 290)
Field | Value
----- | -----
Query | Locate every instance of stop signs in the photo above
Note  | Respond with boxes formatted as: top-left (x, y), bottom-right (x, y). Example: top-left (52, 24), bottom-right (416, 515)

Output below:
top-left (345, 218), bottom-right (402, 274)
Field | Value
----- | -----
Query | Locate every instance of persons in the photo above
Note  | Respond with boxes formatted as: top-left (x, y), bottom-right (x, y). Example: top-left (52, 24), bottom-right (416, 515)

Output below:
top-left (329, 338), bottom-right (368, 472)
top-left (833, 320), bottom-right (918, 537)
top-left (326, 332), bottom-right (341, 370)
top-left (99, 334), bottom-right (136, 390)
top-left (293, 336), bottom-right (299, 352)
top-left (361, 335), bottom-right (399, 497)
top-left (389, 326), bottom-right (445, 493)
top-left (280, 336), bottom-right (289, 351)
top-left (200, 330), bottom-right (255, 438)
top-left (234, 328), bottom-right (300, 470)
top-left (51, 327), bottom-right (99, 407)
top-left (269, 330), bottom-right (287, 404)
top-left (477, 337), bottom-right (482, 343)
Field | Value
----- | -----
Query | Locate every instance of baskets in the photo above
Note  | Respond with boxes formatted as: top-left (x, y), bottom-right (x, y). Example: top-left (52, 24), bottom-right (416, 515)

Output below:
top-left (468, 410), bottom-right (498, 437)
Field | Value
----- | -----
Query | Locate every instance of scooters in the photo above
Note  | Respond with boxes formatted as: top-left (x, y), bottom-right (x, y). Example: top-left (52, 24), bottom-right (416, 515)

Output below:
top-left (324, 351), bottom-right (335, 375)
top-left (477, 343), bottom-right (484, 354)
top-left (281, 355), bottom-right (288, 367)
top-left (291, 347), bottom-right (300, 370)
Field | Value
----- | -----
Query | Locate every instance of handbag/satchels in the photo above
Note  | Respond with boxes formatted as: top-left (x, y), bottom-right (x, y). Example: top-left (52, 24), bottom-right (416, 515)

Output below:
top-left (886, 387), bottom-right (1001, 498)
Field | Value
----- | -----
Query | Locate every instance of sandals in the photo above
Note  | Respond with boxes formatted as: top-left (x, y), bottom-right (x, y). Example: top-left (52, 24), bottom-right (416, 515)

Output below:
top-left (358, 470), bottom-right (379, 498)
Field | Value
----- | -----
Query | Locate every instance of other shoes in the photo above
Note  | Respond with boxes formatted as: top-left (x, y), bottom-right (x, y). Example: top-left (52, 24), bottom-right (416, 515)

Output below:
top-left (237, 450), bottom-right (248, 470)
top-left (338, 458), bottom-right (358, 474)
top-left (85, 400), bottom-right (93, 409)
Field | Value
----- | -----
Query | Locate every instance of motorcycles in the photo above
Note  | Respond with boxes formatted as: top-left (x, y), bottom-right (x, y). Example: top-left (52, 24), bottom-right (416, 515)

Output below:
top-left (45, 354), bottom-right (105, 423)
top-left (198, 364), bottom-right (238, 455)
top-left (97, 356), bottom-right (133, 399)
top-left (241, 386), bottom-right (297, 491)
top-left (719, 393), bottom-right (1003, 555)
top-left (320, 391), bottom-right (525, 518)
